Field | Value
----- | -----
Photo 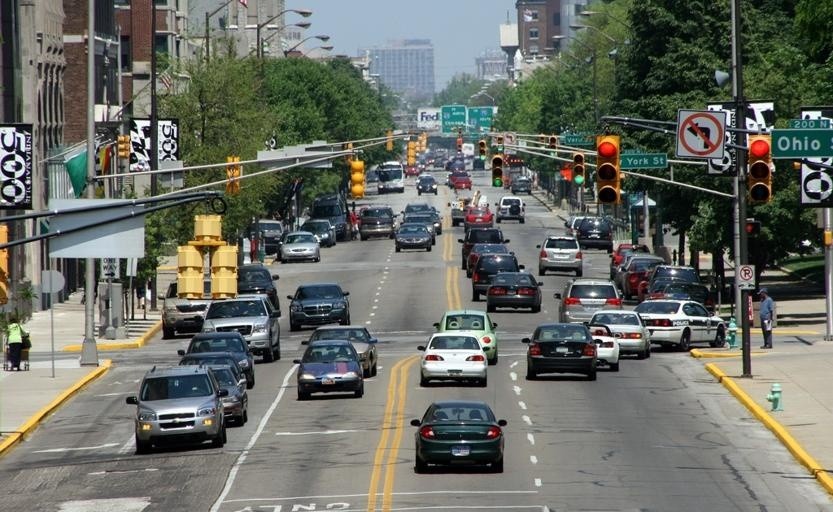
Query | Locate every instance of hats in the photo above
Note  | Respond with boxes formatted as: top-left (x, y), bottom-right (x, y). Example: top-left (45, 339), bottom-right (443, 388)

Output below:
top-left (757, 288), bottom-right (768, 295)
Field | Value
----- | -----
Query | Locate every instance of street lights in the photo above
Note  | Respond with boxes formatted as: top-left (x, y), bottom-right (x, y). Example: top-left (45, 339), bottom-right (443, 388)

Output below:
top-left (303, 44), bottom-right (337, 63)
top-left (256, 18), bottom-right (310, 63)
top-left (199, 0), bottom-right (249, 148)
top-left (282, 34), bottom-right (329, 65)
top-left (243, 9), bottom-right (317, 64)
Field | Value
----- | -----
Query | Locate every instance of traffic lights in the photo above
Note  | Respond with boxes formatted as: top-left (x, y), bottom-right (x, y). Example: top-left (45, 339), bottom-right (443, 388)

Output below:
top-left (594, 140), bottom-right (623, 203)
top-left (491, 155), bottom-right (505, 188)
top-left (743, 219), bottom-right (760, 239)
top-left (480, 141), bottom-right (487, 161)
top-left (540, 134), bottom-right (546, 145)
top-left (572, 154), bottom-right (587, 186)
top-left (749, 138), bottom-right (772, 205)
top-left (495, 138), bottom-right (505, 153)
top-left (549, 136), bottom-right (558, 158)
top-left (456, 136), bottom-right (463, 154)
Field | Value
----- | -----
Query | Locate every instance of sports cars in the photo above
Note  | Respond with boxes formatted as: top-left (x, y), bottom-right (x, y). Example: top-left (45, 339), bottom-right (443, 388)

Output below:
top-left (585, 326), bottom-right (621, 370)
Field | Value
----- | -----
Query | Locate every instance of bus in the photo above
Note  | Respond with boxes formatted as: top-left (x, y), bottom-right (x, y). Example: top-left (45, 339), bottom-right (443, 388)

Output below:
top-left (377, 161), bottom-right (406, 197)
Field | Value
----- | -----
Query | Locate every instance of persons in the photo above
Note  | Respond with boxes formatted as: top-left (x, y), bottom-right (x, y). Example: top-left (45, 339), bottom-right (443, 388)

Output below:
top-left (350, 211), bottom-right (360, 241)
top-left (4, 315), bottom-right (30, 371)
top-left (336, 348), bottom-right (354, 364)
top-left (342, 330), bottom-right (360, 343)
top-left (228, 340), bottom-right (241, 353)
top-left (199, 344), bottom-right (208, 352)
top-left (216, 302), bottom-right (262, 317)
top-left (759, 287), bottom-right (776, 351)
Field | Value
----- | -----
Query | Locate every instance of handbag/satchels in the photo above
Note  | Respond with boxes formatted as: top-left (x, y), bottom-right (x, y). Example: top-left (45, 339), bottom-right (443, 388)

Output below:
top-left (22, 339), bottom-right (32, 349)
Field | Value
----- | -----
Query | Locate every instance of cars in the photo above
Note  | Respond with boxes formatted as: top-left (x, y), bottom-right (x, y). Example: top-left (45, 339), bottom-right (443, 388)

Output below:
top-left (235, 196), bottom-right (439, 262)
top-left (309, 326), bottom-right (379, 375)
top-left (513, 177), bottom-right (532, 194)
top-left (286, 284), bottom-right (352, 328)
top-left (609, 245), bottom-right (705, 306)
top-left (422, 336), bottom-right (488, 386)
top-left (487, 277), bottom-right (544, 313)
top-left (134, 266), bottom-right (280, 452)
top-left (412, 404), bottom-right (508, 476)
top-left (407, 137), bottom-right (476, 201)
top-left (456, 197), bottom-right (538, 297)
top-left (592, 311), bottom-right (654, 361)
top-left (527, 323), bottom-right (598, 377)
top-left (294, 346), bottom-right (366, 399)
top-left (637, 299), bottom-right (731, 347)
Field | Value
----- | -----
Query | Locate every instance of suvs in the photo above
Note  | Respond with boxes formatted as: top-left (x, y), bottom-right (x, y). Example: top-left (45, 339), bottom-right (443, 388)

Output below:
top-left (572, 218), bottom-right (611, 248)
top-left (431, 311), bottom-right (498, 362)
top-left (538, 237), bottom-right (585, 277)
top-left (561, 278), bottom-right (619, 324)
